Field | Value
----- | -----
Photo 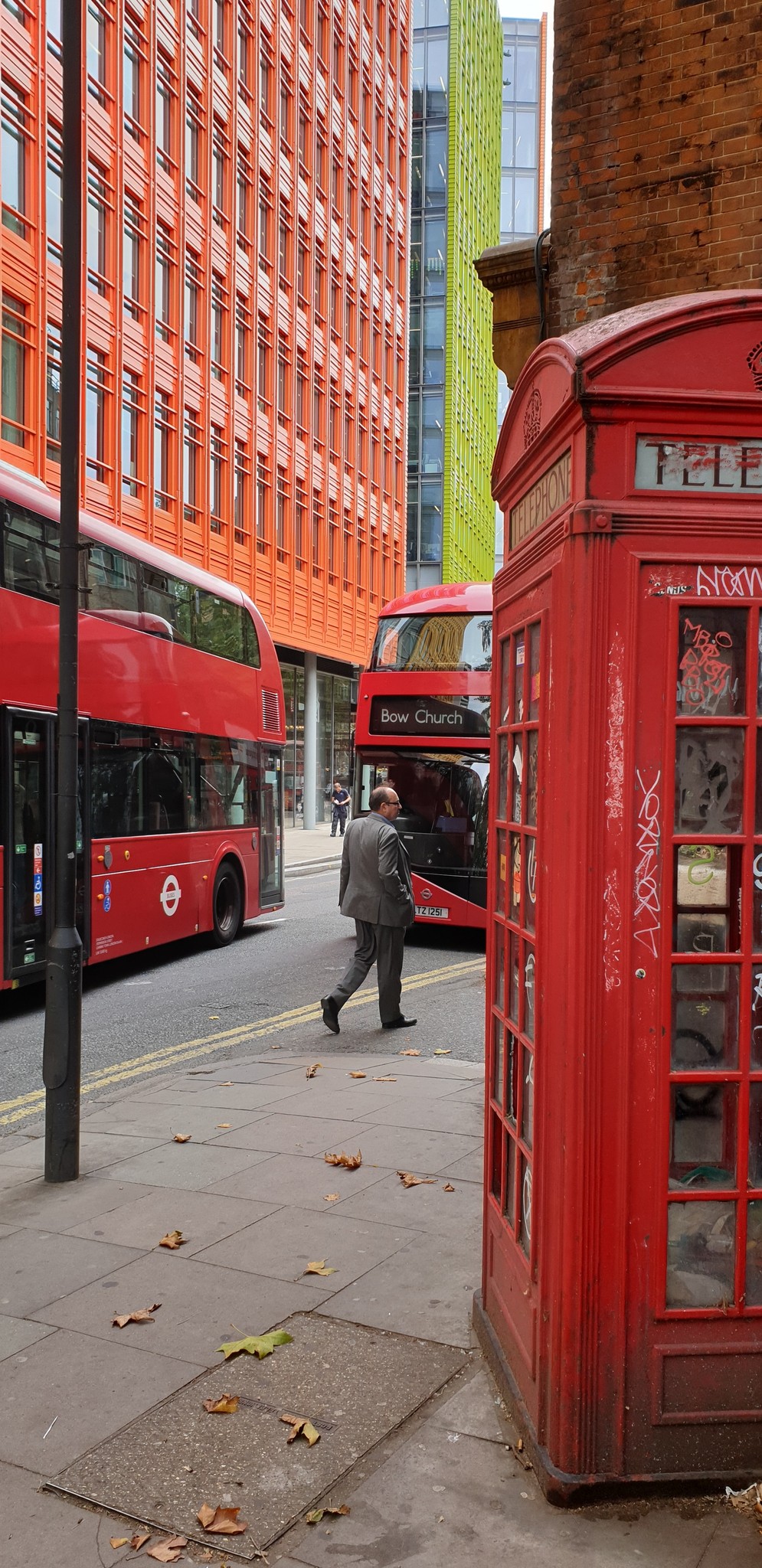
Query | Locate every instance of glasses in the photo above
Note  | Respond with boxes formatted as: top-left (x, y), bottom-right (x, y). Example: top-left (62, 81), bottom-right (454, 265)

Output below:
top-left (379, 801), bottom-right (399, 807)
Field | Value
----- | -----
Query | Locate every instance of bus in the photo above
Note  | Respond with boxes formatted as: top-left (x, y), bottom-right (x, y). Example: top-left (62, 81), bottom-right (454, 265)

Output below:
top-left (2, 461), bottom-right (285, 995)
top-left (349, 581), bottom-right (494, 940)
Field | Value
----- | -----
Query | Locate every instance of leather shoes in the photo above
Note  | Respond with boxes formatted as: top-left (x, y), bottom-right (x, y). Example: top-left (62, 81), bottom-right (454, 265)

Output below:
top-left (382, 1014), bottom-right (417, 1029)
top-left (321, 994), bottom-right (341, 1033)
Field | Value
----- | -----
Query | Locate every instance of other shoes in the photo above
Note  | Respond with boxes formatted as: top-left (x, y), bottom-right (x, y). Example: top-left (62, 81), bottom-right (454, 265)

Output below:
top-left (339, 833), bottom-right (344, 837)
top-left (330, 832), bottom-right (336, 837)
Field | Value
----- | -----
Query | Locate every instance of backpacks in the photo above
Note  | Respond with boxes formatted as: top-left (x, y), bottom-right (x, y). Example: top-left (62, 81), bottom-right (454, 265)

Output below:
top-left (334, 789), bottom-right (350, 806)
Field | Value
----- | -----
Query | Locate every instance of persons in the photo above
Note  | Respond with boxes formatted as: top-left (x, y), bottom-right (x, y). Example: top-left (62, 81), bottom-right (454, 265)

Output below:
top-left (392, 759), bottom-right (475, 832)
top-left (330, 783), bottom-right (351, 837)
top-left (320, 786), bottom-right (417, 1033)
top-left (76, 727), bottom-right (193, 832)
top-left (377, 773), bottom-right (382, 784)
